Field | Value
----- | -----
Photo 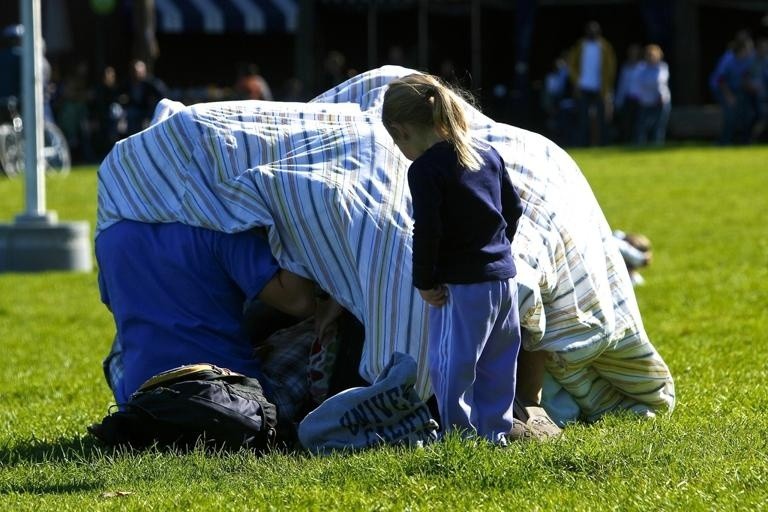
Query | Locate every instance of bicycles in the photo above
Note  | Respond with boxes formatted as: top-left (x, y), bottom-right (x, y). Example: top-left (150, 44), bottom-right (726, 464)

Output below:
top-left (1, 121), bottom-right (69, 180)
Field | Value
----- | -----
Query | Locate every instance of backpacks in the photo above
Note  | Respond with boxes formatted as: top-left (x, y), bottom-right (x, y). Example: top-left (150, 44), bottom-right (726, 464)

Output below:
top-left (87, 362), bottom-right (279, 456)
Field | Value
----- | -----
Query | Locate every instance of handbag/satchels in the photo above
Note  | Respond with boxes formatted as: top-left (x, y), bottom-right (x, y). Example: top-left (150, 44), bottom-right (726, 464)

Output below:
top-left (294, 348), bottom-right (440, 459)
